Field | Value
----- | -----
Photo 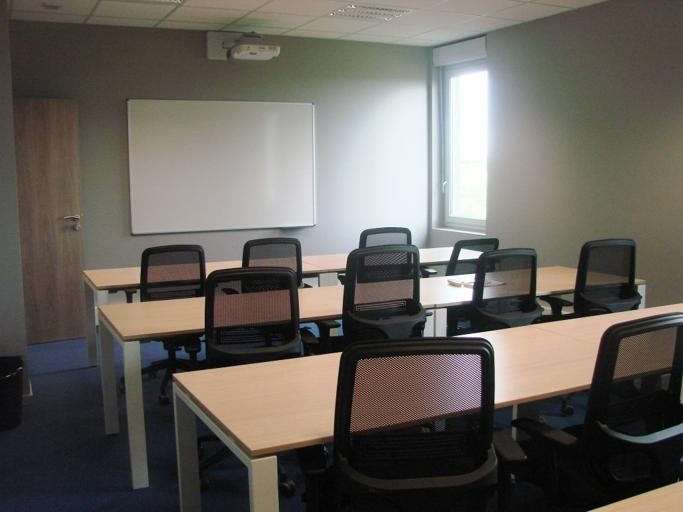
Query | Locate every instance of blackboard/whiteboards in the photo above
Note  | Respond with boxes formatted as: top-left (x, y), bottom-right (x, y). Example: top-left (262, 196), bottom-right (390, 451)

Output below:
top-left (126, 100), bottom-right (316, 236)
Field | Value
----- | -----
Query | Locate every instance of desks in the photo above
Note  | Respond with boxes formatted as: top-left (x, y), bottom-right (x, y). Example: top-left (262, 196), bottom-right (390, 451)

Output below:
top-left (171, 302), bottom-right (682, 512)
top-left (98, 265), bottom-right (645, 491)
top-left (591, 480), bottom-right (681, 511)
top-left (83, 244), bottom-right (487, 394)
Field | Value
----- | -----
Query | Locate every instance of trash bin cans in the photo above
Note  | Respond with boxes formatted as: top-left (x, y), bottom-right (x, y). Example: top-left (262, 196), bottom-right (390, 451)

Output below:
top-left (0, 356), bottom-right (23, 429)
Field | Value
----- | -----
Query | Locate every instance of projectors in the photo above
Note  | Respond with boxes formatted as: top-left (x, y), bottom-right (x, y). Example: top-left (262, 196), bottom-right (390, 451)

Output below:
top-left (227, 38), bottom-right (280, 61)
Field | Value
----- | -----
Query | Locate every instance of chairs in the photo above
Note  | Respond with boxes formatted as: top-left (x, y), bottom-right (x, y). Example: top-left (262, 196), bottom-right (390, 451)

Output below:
top-left (420, 237), bottom-right (500, 279)
top-left (539, 238), bottom-right (644, 321)
top-left (337, 225), bottom-right (413, 284)
top-left (203, 265), bottom-right (318, 369)
top-left (473, 248), bottom-right (544, 333)
top-left (220, 236), bottom-right (314, 349)
top-left (108, 246), bottom-right (213, 392)
top-left (508, 311), bottom-right (682, 503)
top-left (316, 245), bottom-right (431, 354)
top-left (323, 337), bottom-right (527, 510)
